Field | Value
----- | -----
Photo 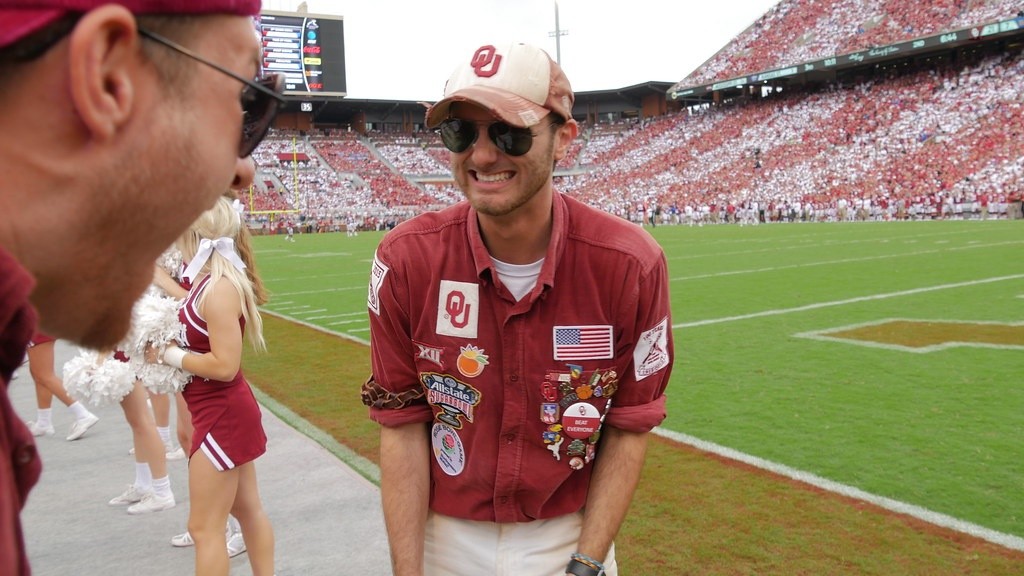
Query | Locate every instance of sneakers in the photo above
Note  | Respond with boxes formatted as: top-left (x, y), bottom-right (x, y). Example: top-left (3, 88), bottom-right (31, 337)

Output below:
top-left (66, 413), bottom-right (99, 441)
top-left (171, 532), bottom-right (194, 547)
top-left (128, 439), bottom-right (173, 455)
top-left (26, 421), bottom-right (55, 437)
top-left (108, 485), bottom-right (150, 506)
top-left (127, 493), bottom-right (175, 515)
top-left (165, 447), bottom-right (186, 460)
top-left (225, 532), bottom-right (247, 558)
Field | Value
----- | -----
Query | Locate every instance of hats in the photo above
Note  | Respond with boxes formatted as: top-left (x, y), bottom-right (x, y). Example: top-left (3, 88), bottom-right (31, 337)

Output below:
top-left (425, 43), bottom-right (575, 129)
top-left (0, 0), bottom-right (262, 47)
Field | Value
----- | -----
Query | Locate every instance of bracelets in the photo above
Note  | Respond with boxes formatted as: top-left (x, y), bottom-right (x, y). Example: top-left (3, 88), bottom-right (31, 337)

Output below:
top-left (572, 557), bottom-right (599, 571)
top-left (164, 346), bottom-right (188, 369)
top-left (565, 559), bottom-right (606, 576)
top-left (572, 553), bottom-right (605, 570)
top-left (158, 344), bottom-right (165, 365)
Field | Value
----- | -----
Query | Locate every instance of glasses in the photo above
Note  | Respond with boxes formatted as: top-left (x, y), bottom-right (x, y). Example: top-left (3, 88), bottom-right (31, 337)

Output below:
top-left (15, 16), bottom-right (288, 160)
top-left (432, 118), bottom-right (562, 156)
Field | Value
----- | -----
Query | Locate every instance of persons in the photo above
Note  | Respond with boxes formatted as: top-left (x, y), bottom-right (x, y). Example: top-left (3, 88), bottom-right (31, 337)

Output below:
top-left (28, 0), bottom-right (1024, 576)
top-left (0, 0), bottom-right (286, 576)
top-left (363, 40), bottom-right (674, 576)
